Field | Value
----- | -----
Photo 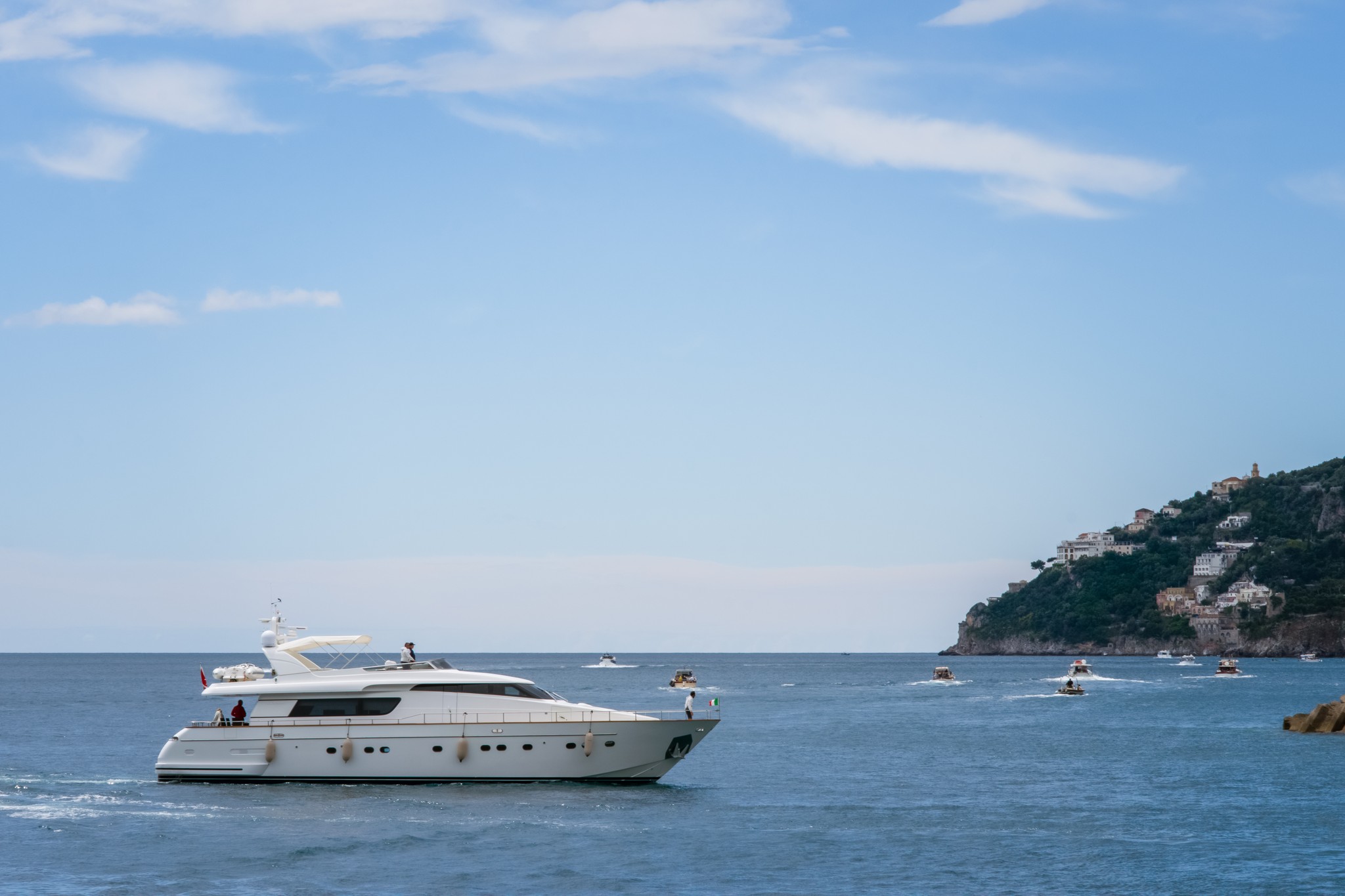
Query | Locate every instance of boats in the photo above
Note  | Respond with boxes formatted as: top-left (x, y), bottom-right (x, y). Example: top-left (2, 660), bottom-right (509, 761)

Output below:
top-left (1180, 655), bottom-right (1195, 664)
top-left (1157, 650), bottom-right (1171, 659)
top-left (841, 653), bottom-right (850, 655)
top-left (1068, 660), bottom-right (1093, 678)
top-left (599, 652), bottom-right (616, 667)
top-left (1054, 685), bottom-right (1086, 696)
top-left (669, 666), bottom-right (697, 688)
top-left (1215, 659), bottom-right (1239, 677)
top-left (1298, 654), bottom-right (1320, 662)
top-left (932, 667), bottom-right (956, 681)
top-left (154, 599), bottom-right (725, 785)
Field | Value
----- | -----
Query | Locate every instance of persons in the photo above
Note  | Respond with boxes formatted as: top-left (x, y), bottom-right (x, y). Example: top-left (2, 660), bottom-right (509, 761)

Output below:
top-left (231, 700), bottom-right (246, 725)
top-left (675, 675), bottom-right (697, 682)
top-left (685, 691), bottom-right (696, 720)
top-left (1219, 662), bottom-right (1222, 666)
top-left (409, 642), bottom-right (415, 668)
top-left (1067, 678), bottom-right (1074, 688)
top-left (1078, 684), bottom-right (1082, 690)
top-left (400, 642), bottom-right (416, 669)
top-left (209, 708), bottom-right (224, 726)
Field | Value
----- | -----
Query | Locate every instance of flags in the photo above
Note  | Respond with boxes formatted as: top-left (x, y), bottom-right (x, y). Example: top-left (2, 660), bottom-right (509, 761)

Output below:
top-left (709, 697), bottom-right (720, 706)
top-left (200, 664), bottom-right (208, 689)
